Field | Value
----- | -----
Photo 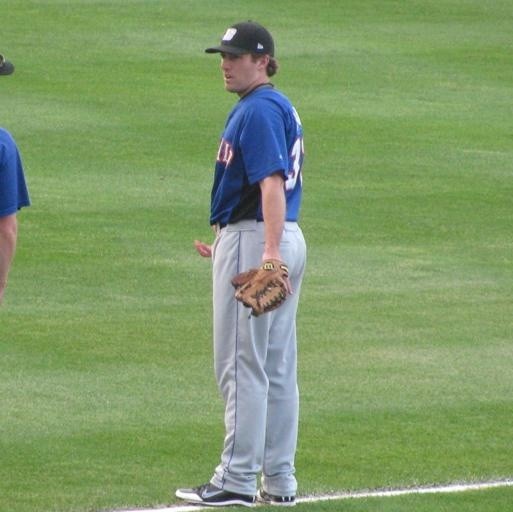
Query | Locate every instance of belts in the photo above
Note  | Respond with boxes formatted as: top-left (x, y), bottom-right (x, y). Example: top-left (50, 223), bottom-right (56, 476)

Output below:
top-left (212, 221), bottom-right (227, 231)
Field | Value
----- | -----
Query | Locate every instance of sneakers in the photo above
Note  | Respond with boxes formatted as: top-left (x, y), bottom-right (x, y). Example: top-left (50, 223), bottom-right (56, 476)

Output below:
top-left (175, 481), bottom-right (297, 508)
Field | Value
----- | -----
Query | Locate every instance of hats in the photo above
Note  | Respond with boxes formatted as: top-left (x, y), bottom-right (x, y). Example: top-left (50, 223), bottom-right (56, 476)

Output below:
top-left (205, 20), bottom-right (273, 55)
top-left (0, 53), bottom-right (14, 76)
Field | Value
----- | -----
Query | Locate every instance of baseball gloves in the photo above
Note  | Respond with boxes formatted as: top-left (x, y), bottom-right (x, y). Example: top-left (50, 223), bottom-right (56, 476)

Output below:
top-left (230, 260), bottom-right (291, 316)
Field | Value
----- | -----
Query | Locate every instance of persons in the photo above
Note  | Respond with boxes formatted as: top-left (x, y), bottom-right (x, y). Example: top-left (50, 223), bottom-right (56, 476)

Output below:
top-left (175, 19), bottom-right (306, 506)
top-left (0, 52), bottom-right (31, 304)
